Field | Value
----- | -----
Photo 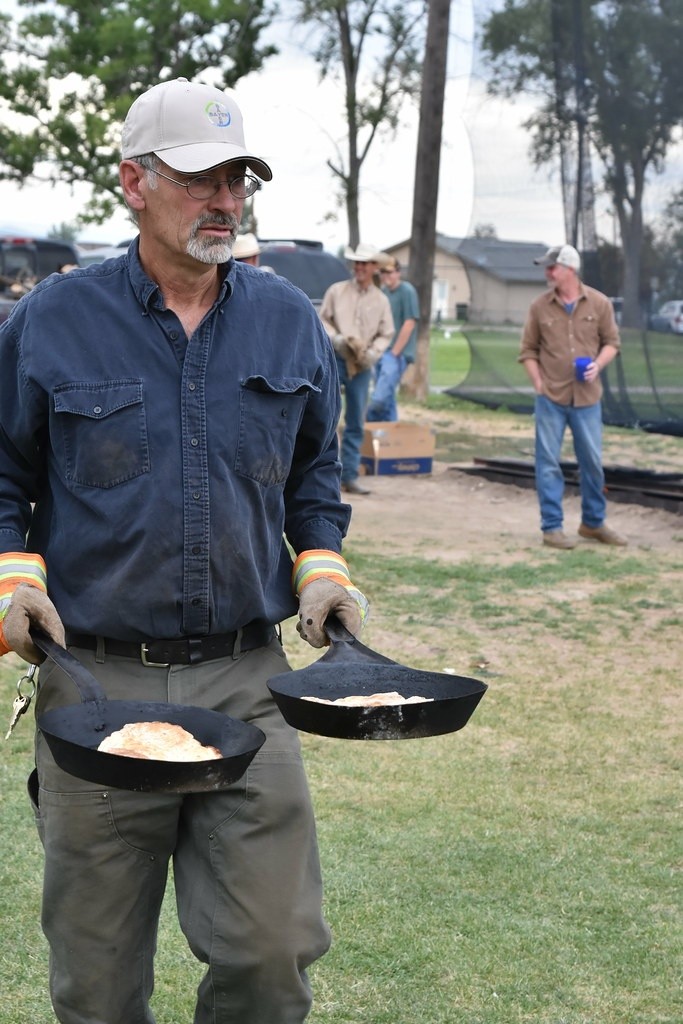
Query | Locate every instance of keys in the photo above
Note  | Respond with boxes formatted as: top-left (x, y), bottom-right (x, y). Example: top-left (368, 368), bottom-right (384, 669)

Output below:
top-left (5, 694), bottom-right (31, 740)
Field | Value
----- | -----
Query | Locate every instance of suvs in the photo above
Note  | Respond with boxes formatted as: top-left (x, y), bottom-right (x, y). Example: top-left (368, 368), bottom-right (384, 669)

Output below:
top-left (69, 235), bottom-right (350, 314)
top-left (0, 234), bottom-right (81, 327)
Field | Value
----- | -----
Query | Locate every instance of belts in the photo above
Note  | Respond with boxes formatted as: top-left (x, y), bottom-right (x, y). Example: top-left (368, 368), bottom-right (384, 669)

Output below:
top-left (65, 623), bottom-right (276, 668)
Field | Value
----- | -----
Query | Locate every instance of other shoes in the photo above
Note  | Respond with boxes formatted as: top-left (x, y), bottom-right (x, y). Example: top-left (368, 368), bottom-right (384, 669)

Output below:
top-left (579, 521), bottom-right (628, 547)
top-left (542, 529), bottom-right (576, 549)
top-left (340, 480), bottom-right (370, 495)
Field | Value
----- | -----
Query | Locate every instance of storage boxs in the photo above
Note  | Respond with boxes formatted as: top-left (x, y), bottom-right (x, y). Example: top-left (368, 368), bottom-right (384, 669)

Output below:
top-left (339, 422), bottom-right (435, 479)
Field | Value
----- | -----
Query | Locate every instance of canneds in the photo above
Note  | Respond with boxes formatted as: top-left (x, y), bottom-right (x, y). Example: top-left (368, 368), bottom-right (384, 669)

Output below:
top-left (574, 356), bottom-right (592, 380)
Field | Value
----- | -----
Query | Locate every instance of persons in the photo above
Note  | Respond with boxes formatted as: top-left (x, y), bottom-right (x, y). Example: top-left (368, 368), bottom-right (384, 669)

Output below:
top-left (515, 243), bottom-right (627, 549)
top-left (317, 242), bottom-right (395, 494)
top-left (367, 261), bottom-right (419, 422)
top-left (0, 76), bottom-right (364, 1023)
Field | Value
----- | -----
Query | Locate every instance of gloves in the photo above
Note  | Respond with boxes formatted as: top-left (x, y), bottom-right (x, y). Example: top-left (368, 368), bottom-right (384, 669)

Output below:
top-left (0, 552), bottom-right (66, 667)
top-left (291, 549), bottom-right (369, 649)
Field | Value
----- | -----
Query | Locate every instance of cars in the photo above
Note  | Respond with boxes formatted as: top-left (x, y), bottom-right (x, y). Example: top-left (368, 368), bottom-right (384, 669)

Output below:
top-left (650, 300), bottom-right (683, 334)
top-left (608, 296), bottom-right (624, 325)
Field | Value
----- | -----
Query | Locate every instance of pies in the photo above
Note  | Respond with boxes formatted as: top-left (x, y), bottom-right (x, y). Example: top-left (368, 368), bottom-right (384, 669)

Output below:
top-left (97, 721), bottom-right (222, 762)
top-left (301, 692), bottom-right (431, 707)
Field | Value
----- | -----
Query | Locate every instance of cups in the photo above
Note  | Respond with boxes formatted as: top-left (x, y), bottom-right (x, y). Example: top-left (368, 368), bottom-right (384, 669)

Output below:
top-left (574, 357), bottom-right (593, 382)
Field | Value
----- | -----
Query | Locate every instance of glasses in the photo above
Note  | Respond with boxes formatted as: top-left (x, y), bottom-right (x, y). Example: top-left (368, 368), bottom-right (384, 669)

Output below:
top-left (140, 163), bottom-right (262, 200)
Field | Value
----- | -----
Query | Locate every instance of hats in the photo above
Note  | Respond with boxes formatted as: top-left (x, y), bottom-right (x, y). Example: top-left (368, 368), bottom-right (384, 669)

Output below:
top-left (230, 233), bottom-right (262, 259)
top-left (378, 258), bottom-right (400, 273)
top-left (121, 77), bottom-right (273, 182)
top-left (533, 243), bottom-right (581, 274)
top-left (343, 243), bottom-right (391, 262)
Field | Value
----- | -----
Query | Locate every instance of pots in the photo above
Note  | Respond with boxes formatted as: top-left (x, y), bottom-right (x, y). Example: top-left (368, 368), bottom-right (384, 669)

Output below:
top-left (265, 613), bottom-right (489, 741)
top-left (28, 619), bottom-right (267, 794)
